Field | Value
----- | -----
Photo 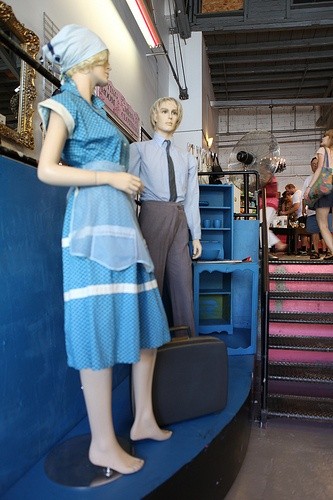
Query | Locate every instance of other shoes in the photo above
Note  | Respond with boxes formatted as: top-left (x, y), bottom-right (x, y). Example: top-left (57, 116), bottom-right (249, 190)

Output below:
top-left (323, 253), bottom-right (333, 260)
top-left (299, 251), bottom-right (308, 256)
top-left (319, 249), bottom-right (326, 254)
top-left (259, 253), bottom-right (277, 260)
top-left (275, 243), bottom-right (287, 251)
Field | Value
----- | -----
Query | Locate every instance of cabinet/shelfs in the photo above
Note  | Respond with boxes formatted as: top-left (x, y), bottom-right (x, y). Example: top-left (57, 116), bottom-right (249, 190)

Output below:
top-left (189, 185), bottom-right (259, 356)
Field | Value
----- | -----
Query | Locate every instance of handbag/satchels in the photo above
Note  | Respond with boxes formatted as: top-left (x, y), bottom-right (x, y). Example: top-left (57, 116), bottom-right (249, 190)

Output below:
top-left (308, 146), bottom-right (332, 198)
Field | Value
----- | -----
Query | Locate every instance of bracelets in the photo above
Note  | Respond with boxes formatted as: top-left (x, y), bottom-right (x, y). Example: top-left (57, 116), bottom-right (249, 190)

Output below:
top-left (308, 185), bottom-right (311, 188)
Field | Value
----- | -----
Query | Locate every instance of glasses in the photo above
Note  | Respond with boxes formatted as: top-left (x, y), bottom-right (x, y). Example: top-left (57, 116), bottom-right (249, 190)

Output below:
top-left (312, 163), bottom-right (318, 166)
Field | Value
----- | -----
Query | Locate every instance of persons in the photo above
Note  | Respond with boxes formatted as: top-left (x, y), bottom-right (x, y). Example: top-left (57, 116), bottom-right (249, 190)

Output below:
top-left (240, 156), bottom-right (328, 259)
top-left (129, 96), bottom-right (204, 336)
top-left (304, 129), bottom-right (333, 260)
top-left (35, 25), bottom-right (175, 475)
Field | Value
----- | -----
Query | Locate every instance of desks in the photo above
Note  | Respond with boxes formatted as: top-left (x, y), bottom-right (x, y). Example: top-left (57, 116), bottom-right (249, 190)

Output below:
top-left (268, 228), bottom-right (294, 255)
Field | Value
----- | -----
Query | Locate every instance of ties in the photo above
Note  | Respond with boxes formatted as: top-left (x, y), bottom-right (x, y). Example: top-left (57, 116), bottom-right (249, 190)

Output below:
top-left (164, 140), bottom-right (177, 202)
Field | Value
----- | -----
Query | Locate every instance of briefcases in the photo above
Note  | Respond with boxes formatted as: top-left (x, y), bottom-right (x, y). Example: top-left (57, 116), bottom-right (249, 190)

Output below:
top-left (130, 326), bottom-right (228, 429)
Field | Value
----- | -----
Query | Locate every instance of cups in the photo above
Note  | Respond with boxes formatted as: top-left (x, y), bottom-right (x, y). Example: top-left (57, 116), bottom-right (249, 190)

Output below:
top-left (204, 219), bottom-right (210, 228)
top-left (213, 219), bottom-right (221, 228)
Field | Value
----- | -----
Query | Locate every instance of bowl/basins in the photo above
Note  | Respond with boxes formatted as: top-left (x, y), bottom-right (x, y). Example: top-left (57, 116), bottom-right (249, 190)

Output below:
top-left (200, 249), bottom-right (220, 260)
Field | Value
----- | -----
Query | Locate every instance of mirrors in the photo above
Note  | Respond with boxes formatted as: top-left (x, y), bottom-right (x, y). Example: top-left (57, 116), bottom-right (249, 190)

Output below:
top-left (0, 1), bottom-right (40, 150)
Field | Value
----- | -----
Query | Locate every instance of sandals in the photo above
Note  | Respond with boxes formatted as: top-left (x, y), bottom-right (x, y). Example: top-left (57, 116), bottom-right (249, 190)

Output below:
top-left (310, 252), bottom-right (319, 259)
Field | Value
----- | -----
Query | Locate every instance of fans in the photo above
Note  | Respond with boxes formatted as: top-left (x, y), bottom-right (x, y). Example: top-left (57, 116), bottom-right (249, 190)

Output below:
top-left (228, 131), bottom-right (280, 221)
